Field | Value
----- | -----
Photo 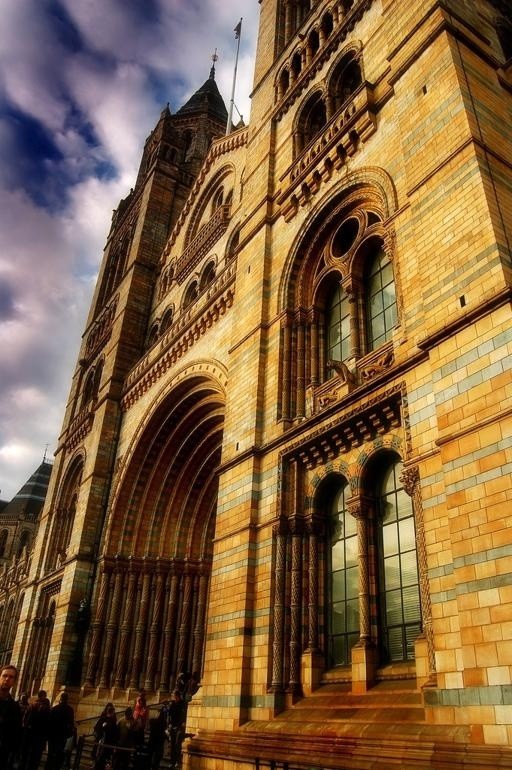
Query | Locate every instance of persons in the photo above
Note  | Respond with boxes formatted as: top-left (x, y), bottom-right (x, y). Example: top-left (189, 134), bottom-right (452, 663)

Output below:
top-left (0, 665), bottom-right (201, 770)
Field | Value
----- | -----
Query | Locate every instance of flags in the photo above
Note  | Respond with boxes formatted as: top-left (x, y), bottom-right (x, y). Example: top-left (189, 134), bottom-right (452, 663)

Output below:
top-left (234, 20), bottom-right (241, 40)
